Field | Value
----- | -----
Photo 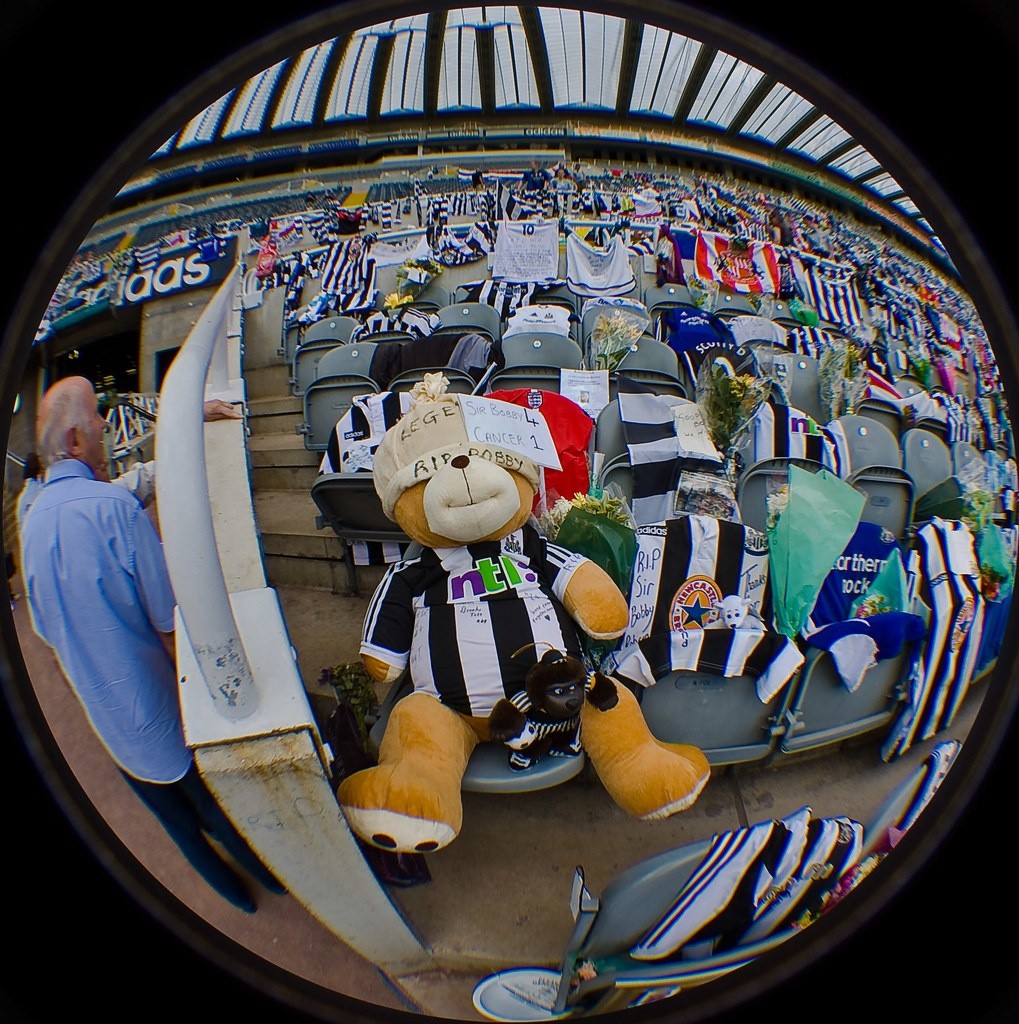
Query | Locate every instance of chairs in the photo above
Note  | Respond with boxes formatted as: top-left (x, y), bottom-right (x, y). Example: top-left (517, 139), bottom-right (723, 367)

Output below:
top-left (275, 283), bottom-right (1018, 1017)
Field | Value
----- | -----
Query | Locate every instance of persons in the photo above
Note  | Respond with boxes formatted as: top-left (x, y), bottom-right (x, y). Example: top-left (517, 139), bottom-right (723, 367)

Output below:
top-left (16, 455), bottom-right (45, 534)
top-left (94, 454), bottom-right (155, 509)
top-left (20, 376), bottom-right (288, 914)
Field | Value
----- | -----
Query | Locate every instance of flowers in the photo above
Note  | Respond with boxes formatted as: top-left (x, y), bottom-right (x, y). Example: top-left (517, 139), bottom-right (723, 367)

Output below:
top-left (537, 490), bottom-right (630, 542)
top-left (964, 493), bottom-right (996, 528)
top-left (315, 660), bottom-right (380, 752)
top-left (856, 593), bottom-right (888, 618)
top-left (688, 277), bottom-right (719, 309)
top-left (764, 483), bottom-right (789, 532)
top-left (980, 565), bottom-right (1004, 599)
top-left (591, 309), bottom-right (643, 369)
top-left (394, 260), bottom-right (444, 302)
top-left (705, 361), bottom-right (764, 451)
top-left (906, 345), bottom-right (931, 367)
top-left (843, 344), bottom-right (861, 380)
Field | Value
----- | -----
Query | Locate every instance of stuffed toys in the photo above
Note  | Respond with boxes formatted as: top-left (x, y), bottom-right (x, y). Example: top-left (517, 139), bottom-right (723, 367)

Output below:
top-left (337, 397), bottom-right (711, 852)
top-left (487, 648), bottom-right (619, 771)
top-left (712, 597), bottom-right (764, 630)
